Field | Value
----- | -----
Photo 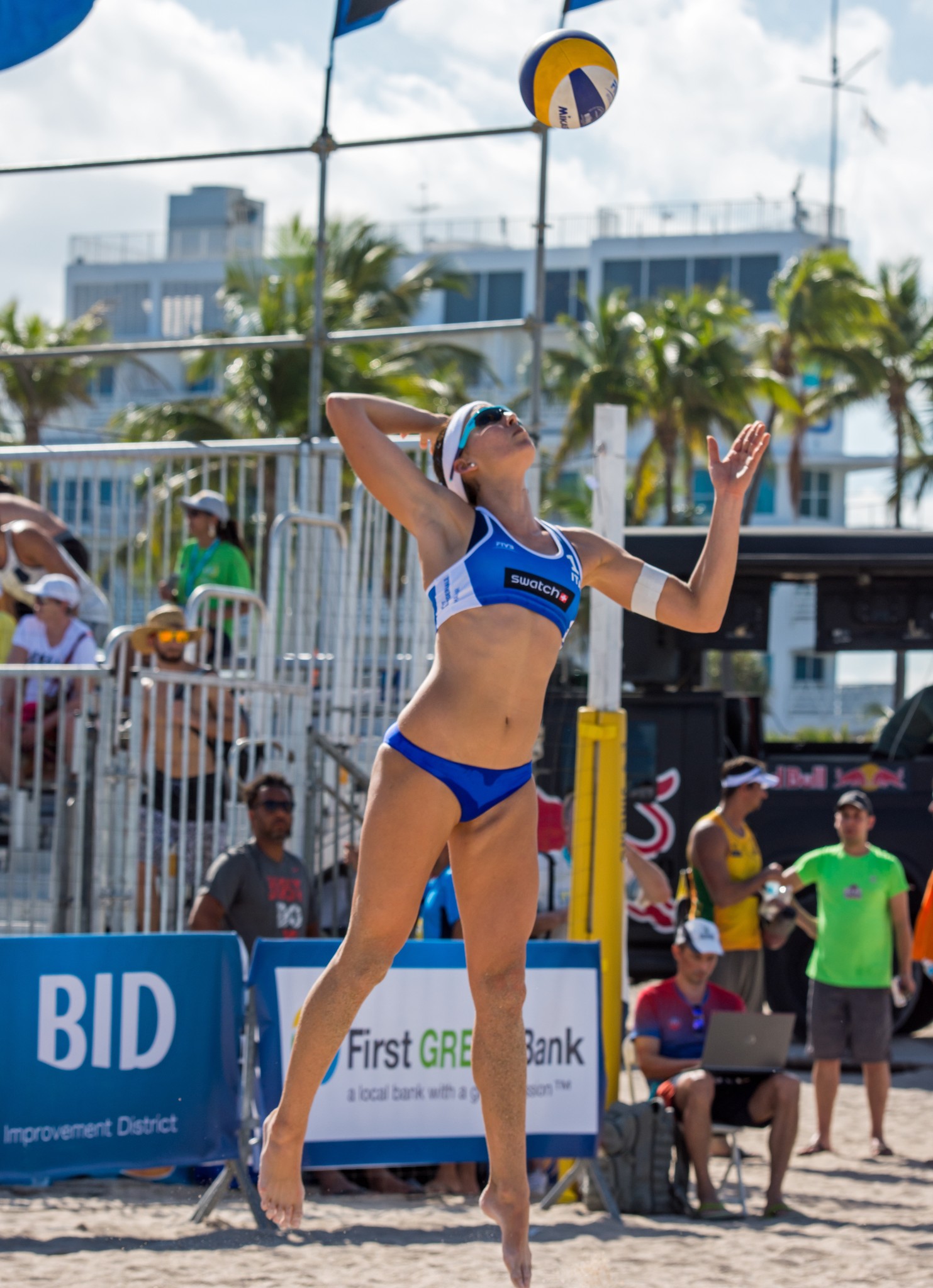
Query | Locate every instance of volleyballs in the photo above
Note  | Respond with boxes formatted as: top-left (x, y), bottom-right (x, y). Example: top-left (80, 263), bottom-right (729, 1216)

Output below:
top-left (517, 27), bottom-right (619, 130)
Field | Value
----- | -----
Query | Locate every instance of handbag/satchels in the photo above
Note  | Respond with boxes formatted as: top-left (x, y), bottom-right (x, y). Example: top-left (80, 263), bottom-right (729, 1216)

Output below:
top-left (20, 631), bottom-right (92, 772)
top-left (173, 664), bottom-right (265, 783)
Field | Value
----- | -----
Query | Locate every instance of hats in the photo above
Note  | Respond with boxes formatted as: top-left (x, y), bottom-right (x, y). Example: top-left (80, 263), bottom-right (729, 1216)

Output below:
top-left (720, 765), bottom-right (780, 790)
top-left (836, 790), bottom-right (873, 812)
top-left (24, 573), bottom-right (82, 610)
top-left (674, 918), bottom-right (724, 957)
top-left (130, 604), bottom-right (205, 655)
top-left (179, 488), bottom-right (230, 528)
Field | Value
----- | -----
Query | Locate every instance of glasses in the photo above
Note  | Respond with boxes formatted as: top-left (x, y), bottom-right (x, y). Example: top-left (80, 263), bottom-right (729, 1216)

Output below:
top-left (449, 405), bottom-right (523, 480)
top-left (255, 800), bottom-right (295, 814)
top-left (153, 631), bottom-right (192, 644)
top-left (184, 508), bottom-right (214, 518)
top-left (34, 595), bottom-right (62, 606)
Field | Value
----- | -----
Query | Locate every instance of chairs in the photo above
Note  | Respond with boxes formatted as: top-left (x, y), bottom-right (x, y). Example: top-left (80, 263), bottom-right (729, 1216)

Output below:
top-left (621, 1031), bottom-right (748, 1223)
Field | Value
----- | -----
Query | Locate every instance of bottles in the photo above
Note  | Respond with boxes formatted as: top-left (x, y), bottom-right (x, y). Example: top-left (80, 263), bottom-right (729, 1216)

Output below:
top-left (12, 789), bottom-right (30, 850)
top-left (762, 879), bottom-right (781, 915)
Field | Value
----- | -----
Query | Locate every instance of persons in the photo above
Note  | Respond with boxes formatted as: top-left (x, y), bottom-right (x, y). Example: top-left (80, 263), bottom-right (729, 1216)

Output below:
top-left (771, 791), bottom-right (917, 1158)
top-left (625, 919), bottom-right (810, 1223)
top-left (255, 391), bottom-right (771, 1288)
top-left (0, 471), bottom-right (671, 1199)
top-left (673, 755), bottom-right (797, 1012)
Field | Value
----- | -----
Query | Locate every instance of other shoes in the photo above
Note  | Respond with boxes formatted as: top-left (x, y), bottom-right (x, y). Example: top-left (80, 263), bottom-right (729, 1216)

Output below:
top-left (870, 1143), bottom-right (892, 1155)
top-left (527, 1172), bottom-right (549, 1203)
top-left (795, 1140), bottom-right (831, 1156)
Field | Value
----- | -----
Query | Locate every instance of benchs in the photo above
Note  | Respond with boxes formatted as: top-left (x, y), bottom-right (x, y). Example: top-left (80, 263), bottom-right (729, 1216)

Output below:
top-left (0, 652), bottom-right (434, 899)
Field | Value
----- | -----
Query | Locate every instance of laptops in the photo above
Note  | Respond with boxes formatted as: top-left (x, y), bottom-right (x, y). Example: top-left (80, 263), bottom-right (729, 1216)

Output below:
top-left (680, 1011), bottom-right (794, 1076)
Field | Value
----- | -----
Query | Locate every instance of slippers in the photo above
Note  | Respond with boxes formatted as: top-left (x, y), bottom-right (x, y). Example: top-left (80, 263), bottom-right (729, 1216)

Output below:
top-left (761, 1203), bottom-right (805, 1224)
top-left (699, 1203), bottom-right (745, 1222)
